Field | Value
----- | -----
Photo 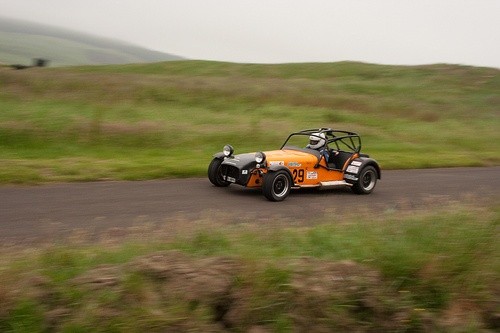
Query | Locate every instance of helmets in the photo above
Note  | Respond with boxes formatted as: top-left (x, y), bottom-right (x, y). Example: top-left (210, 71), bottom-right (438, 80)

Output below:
top-left (309, 132), bottom-right (326, 150)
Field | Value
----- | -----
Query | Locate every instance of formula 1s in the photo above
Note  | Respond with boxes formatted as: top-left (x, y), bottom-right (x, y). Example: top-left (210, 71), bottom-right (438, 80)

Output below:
top-left (207, 128), bottom-right (383, 202)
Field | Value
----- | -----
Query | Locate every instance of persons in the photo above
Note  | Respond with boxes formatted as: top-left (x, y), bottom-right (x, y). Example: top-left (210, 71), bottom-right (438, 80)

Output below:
top-left (304, 132), bottom-right (329, 166)
